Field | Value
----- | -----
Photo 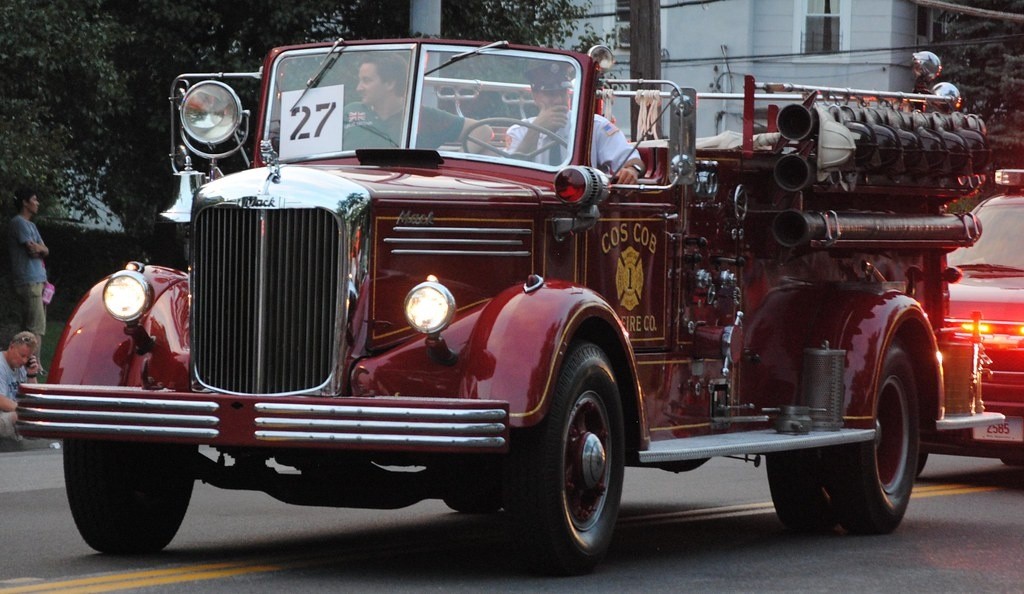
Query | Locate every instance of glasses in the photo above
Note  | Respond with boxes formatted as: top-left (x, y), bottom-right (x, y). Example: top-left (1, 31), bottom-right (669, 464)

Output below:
top-left (13, 336), bottom-right (33, 344)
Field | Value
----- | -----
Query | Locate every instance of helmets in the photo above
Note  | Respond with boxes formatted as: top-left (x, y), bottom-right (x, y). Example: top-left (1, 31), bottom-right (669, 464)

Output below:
top-left (812, 103), bottom-right (991, 181)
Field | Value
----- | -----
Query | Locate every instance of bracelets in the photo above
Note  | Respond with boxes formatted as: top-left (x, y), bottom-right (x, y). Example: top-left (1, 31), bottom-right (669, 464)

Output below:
top-left (26, 373), bottom-right (38, 378)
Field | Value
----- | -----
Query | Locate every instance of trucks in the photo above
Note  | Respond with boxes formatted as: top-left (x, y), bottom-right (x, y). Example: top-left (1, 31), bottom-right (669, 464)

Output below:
top-left (14, 39), bottom-right (991, 574)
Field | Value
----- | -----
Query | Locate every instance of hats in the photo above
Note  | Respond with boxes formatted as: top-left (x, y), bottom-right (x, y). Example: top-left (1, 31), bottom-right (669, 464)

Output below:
top-left (525, 61), bottom-right (576, 90)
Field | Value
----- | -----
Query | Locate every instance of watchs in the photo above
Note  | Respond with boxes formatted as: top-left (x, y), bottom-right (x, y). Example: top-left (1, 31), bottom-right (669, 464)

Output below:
top-left (632, 165), bottom-right (643, 174)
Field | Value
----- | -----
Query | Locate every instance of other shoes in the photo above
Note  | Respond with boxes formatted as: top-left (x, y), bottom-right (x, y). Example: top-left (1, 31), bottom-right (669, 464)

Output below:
top-left (36, 369), bottom-right (47, 376)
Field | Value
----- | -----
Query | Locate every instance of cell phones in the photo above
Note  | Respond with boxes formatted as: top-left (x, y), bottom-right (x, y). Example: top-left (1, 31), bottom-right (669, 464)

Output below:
top-left (26, 357), bottom-right (32, 367)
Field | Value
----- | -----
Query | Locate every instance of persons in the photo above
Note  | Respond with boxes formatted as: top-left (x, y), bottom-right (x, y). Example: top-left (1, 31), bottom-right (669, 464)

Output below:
top-left (8, 188), bottom-right (51, 377)
top-left (0, 331), bottom-right (39, 441)
top-left (347, 229), bottom-right (363, 321)
top-left (343, 53), bottom-right (494, 153)
top-left (506, 62), bottom-right (646, 197)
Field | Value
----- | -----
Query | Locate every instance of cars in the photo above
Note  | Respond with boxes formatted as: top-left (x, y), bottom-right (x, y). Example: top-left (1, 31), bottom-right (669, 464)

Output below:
top-left (918, 167), bottom-right (1024, 476)
